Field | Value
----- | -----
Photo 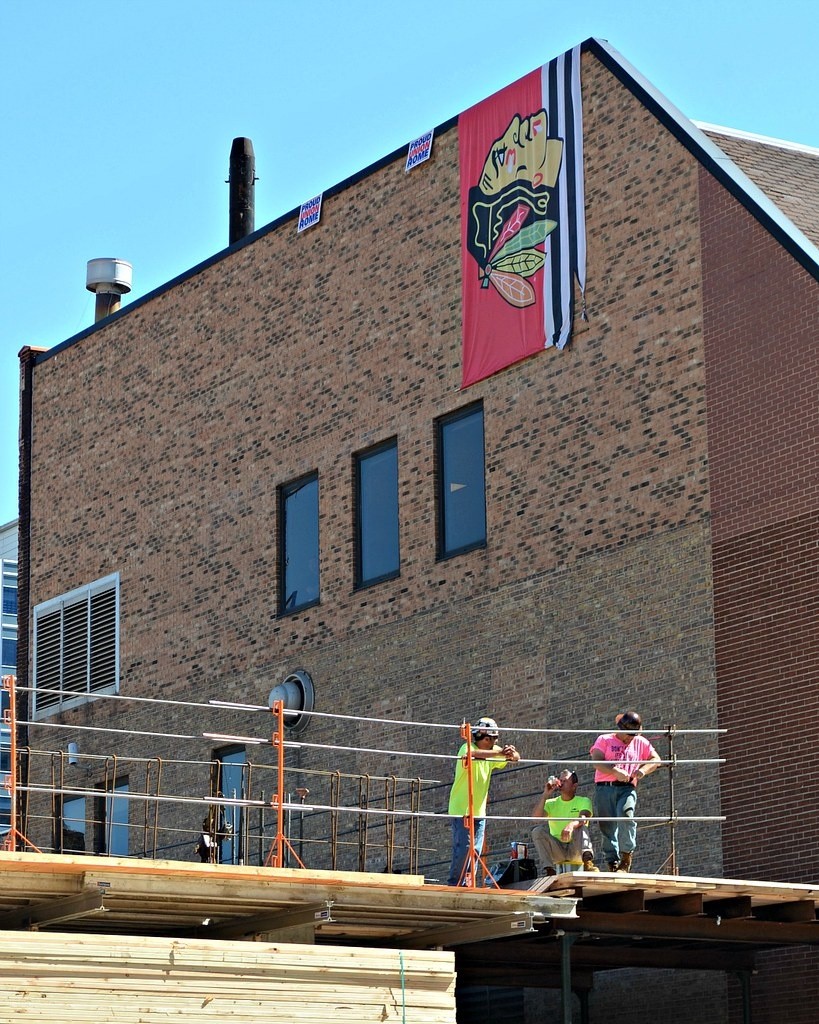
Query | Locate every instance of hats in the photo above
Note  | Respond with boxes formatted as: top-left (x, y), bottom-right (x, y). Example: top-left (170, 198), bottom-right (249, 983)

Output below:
top-left (471, 717), bottom-right (499, 736)
top-left (615, 712), bottom-right (643, 736)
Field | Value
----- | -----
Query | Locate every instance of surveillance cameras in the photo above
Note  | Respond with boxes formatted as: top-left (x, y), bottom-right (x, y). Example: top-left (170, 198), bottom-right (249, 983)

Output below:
top-left (68, 743), bottom-right (78, 765)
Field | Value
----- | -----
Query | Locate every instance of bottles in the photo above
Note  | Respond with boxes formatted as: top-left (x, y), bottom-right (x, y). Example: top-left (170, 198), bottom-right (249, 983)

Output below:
top-left (466, 865), bottom-right (471, 886)
top-left (548, 776), bottom-right (562, 789)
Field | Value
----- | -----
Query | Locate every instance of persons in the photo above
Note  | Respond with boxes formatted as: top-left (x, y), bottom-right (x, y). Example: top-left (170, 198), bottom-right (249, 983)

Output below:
top-left (448, 717), bottom-right (520, 886)
top-left (531, 767), bottom-right (599, 876)
top-left (589, 711), bottom-right (661, 873)
top-left (194, 818), bottom-right (229, 864)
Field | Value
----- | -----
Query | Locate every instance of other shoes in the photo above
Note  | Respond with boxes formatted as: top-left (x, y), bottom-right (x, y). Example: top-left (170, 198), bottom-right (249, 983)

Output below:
top-left (583, 864), bottom-right (600, 872)
top-left (542, 870), bottom-right (556, 876)
top-left (608, 861), bottom-right (632, 873)
